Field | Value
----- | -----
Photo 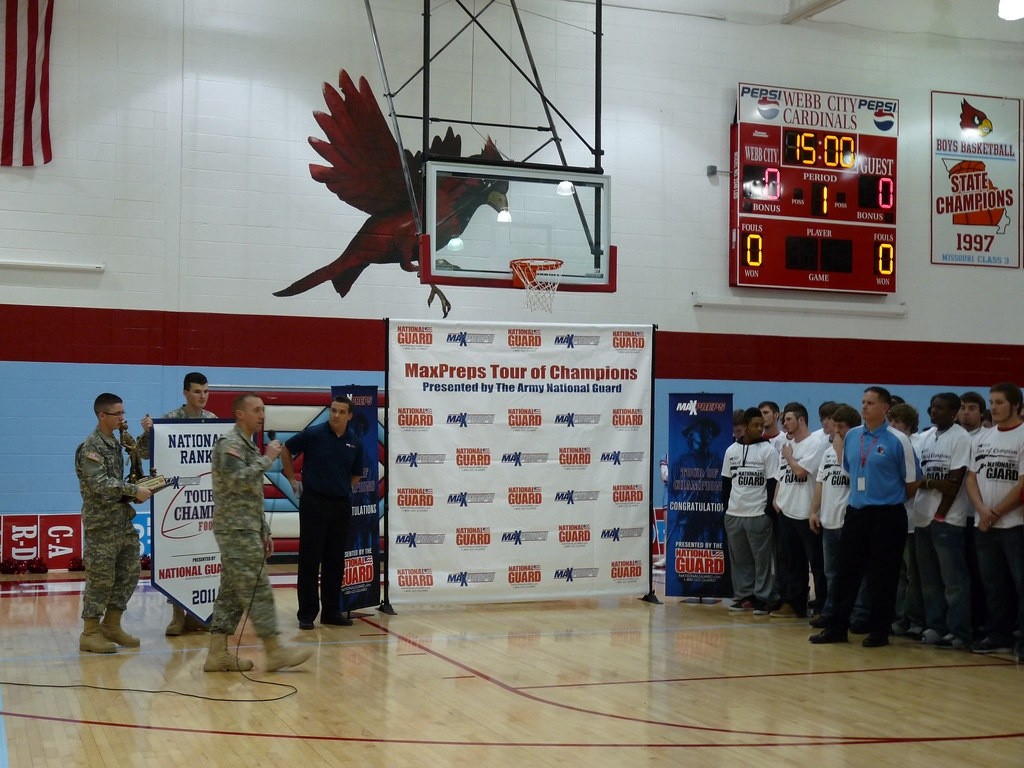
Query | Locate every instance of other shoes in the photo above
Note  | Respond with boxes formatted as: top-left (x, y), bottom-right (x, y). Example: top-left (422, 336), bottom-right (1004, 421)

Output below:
top-left (321, 615), bottom-right (354, 626)
top-left (728, 595), bottom-right (829, 624)
top-left (1014, 642), bottom-right (1024, 665)
top-left (299, 618), bottom-right (314, 630)
top-left (809, 625), bottom-right (848, 644)
top-left (892, 619), bottom-right (1013, 653)
top-left (863, 630), bottom-right (889, 647)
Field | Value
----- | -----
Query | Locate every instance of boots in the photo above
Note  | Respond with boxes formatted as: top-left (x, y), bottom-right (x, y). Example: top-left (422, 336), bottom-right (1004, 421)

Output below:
top-left (182, 613), bottom-right (212, 631)
top-left (165, 604), bottom-right (185, 634)
top-left (262, 634), bottom-right (314, 671)
top-left (202, 632), bottom-right (254, 670)
top-left (79, 618), bottom-right (119, 652)
top-left (99, 607), bottom-right (140, 647)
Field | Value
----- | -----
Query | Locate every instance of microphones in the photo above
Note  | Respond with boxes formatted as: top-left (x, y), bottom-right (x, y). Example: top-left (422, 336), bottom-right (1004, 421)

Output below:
top-left (268, 430), bottom-right (280, 458)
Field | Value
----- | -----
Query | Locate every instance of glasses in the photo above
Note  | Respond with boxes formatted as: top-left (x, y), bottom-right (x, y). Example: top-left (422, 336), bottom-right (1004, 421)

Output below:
top-left (96, 411), bottom-right (126, 417)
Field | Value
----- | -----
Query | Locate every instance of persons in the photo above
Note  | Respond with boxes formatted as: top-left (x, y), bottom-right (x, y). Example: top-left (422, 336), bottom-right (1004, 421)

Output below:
top-left (282, 397), bottom-right (362, 630)
top-left (135, 372), bottom-right (219, 459)
top-left (723, 384), bottom-right (1024, 663)
top-left (75, 393), bottom-right (151, 653)
top-left (205, 394), bottom-right (311, 671)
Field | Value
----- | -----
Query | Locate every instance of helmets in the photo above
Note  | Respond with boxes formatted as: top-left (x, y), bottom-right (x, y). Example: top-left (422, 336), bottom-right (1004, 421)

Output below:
top-left (2, 554), bottom-right (153, 575)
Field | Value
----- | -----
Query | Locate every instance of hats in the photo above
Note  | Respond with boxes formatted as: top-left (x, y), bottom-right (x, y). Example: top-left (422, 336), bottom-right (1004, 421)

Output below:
top-left (682, 415), bottom-right (721, 438)
